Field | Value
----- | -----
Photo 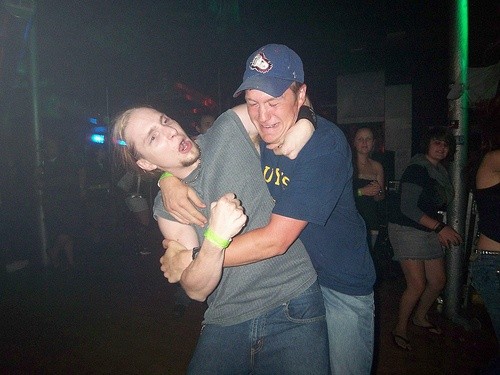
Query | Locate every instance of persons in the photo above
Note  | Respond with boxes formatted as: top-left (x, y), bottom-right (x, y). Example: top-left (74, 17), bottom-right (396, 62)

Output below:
top-left (386, 123), bottom-right (462, 351)
top-left (110, 96), bottom-right (330, 375)
top-left (41, 140), bottom-right (157, 268)
top-left (159, 44), bottom-right (378, 375)
top-left (466, 101), bottom-right (500, 343)
top-left (352, 126), bottom-right (385, 254)
top-left (194, 113), bottom-right (215, 134)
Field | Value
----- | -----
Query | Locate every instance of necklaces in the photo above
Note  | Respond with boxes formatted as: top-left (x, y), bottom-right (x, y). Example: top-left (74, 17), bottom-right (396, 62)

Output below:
top-left (177, 144), bottom-right (202, 183)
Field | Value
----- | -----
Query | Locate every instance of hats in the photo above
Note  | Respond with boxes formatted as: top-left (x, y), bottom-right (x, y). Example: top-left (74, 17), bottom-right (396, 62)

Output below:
top-left (233, 42), bottom-right (304, 98)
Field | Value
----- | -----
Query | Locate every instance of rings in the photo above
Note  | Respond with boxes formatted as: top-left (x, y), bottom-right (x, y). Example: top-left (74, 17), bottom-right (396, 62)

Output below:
top-left (379, 190), bottom-right (382, 193)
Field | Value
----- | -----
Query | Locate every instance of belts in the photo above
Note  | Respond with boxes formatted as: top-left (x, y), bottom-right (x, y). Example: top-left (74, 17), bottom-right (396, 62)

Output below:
top-left (473, 249), bottom-right (500, 255)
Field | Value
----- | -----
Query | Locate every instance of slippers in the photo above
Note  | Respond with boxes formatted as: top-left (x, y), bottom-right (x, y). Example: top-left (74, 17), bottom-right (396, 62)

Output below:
top-left (390, 329), bottom-right (413, 352)
top-left (411, 317), bottom-right (444, 336)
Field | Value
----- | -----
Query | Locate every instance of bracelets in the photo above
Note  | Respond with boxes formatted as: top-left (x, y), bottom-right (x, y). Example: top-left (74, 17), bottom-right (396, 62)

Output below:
top-left (192, 247), bottom-right (200, 260)
top-left (204, 227), bottom-right (231, 249)
top-left (157, 171), bottom-right (175, 187)
top-left (434, 223), bottom-right (447, 233)
top-left (432, 223), bottom-right (440, 230)
top-left (295, 105), bottom-right (317, 129)
top-left (356, 188), bottom-right (362, 196)
